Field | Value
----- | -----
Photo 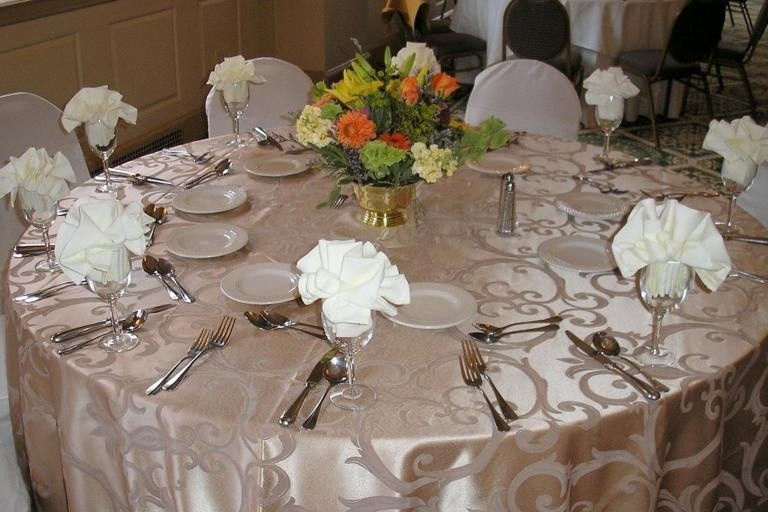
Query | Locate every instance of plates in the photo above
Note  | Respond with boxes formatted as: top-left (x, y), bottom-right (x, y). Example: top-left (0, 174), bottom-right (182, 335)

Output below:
top-left (536, 235), bottom-right (619, 274)
top-left (465, 152), bottom-right (529, 174)
top-left (380, 282), bottom-right (477, 329)
top-left (555, 192), bottom-right (625, 219)
top-left (165, 155), bottom-right (310, 304)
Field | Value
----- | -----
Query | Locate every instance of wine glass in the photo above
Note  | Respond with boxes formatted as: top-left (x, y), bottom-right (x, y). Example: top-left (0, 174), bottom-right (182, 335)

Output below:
top-left (86, 119), bottom-right (124, 193)
top-left (712, 157), bottom-right (758, 238)
top-left (593, 95), bottom-right (624, 164)
top-left (634, 262), bottom-right (692, 371)
top-left (322, 310), bottom-right (375, 409)
top-left (22, 188), bottom-right (66, 274)
top-left (223, 81), bottom-right (252, 148)
top-left (85, 245), bottom-right (140, 353)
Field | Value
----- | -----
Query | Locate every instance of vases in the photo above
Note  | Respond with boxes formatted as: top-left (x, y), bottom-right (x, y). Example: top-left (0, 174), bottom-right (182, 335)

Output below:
top-left (351, 183), bottom-right (416, 228)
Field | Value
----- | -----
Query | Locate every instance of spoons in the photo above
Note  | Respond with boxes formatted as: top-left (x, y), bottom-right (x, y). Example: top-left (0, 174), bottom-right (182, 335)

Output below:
top-left (594, 330), bottom-right (671, 395)
top-left (303, 355), bottom-right (354, 429)
top-left (142, 255), bottom-right (195, 303)
top-left (58, 309), bottom-right (148, 357)
top-left (470, 316), bottom-right (565, 343)
top-left (244, 307), bottom-right (340, 341)
top-left (145, 204), bottom-right (167, 248)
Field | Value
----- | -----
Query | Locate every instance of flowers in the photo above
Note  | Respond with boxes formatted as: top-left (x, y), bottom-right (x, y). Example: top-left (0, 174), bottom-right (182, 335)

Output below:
top-left (290, 44), bottom-right (514, 209)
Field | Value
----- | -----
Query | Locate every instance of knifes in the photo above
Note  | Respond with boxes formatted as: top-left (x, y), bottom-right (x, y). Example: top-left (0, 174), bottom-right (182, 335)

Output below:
top-left (278, 337), bottom-right (337, 428)
top-left (565, 329), bottom-right (660, 406)
top-left (105, 168), bottom-right (172, 188)
top-left (50, 305), bottom-right (178, 342)
top-left (254, 126), bottom-right (286, 152)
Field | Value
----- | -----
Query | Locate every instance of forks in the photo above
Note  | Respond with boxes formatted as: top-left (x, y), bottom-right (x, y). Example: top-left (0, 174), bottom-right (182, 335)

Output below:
top-left (640, 190), bottom-right (718, 201)
top-left (144, 315), bottom-right (236, 395)
top-left (572, 173), bottom-right (629, 193)
top-left (163, 151), bottom-right (215, 164)
top-left (329, 195), bottom-right (348, 209)
top-left (459, 338), bottom-right (519, 432)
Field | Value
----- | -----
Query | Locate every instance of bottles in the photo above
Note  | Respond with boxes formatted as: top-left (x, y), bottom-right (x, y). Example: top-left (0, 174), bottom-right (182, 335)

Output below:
top-left (496, 172), bottom-right (515, 238)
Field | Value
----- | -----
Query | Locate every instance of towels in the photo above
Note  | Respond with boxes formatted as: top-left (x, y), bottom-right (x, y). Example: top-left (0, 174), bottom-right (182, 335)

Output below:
top-left (585, 65), bottom-right (639, 122)
top-left (1, 148), bottom-right (77, 210)
top-left (207, 54), bottom-right (266, 107)
top-left (61, 83), bottom-right (138, 148)
top-left (702, 115), bottom-right (768, 186)
top-left (298, 238), bottom-right (413, 337)
top-left (612, 197), bottom-right (734, 300)
top-left (392, 42), bottom-right (444, 76)
top-left (54, 196), bottom-right (158, 282)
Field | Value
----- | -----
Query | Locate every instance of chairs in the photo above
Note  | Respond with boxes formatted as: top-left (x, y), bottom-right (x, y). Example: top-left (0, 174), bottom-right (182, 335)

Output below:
top-left (205, 57), bottom-right (316, 138)
top-left (0, 92), bottom-right (91, 314)
top-left (681, 0), bottom-right (768, 120)
top-left (490, 0), bottom-right (582, 98)
top-left (384, 3), bottom-right (485, 102)
top-left (725, 0), bottom-right (754, 39)
top-left (618, 0), bottom-right (729, 154)
top-left (464, 59), bottom-right (583, 141)
